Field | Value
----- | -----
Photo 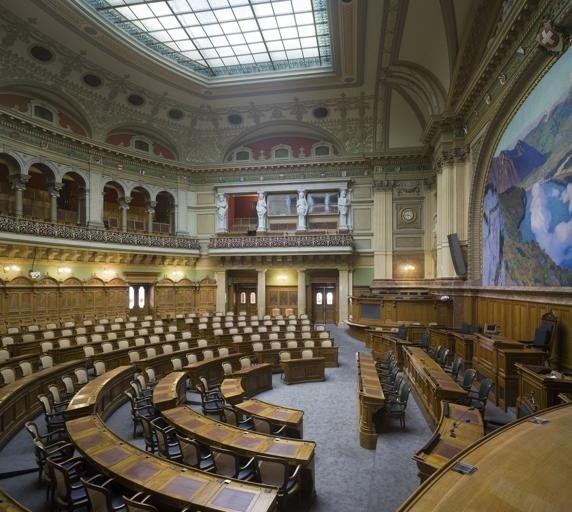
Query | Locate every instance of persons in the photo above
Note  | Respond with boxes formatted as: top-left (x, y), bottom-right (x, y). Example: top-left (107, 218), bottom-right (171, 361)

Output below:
top-left (216, 195), bottom-right (227, 217)
top-left (337, 191), bottom-right (351, 216)
top-left (256, 193), bottom-right (266, 214)
top-left (295, 192), bottom-right (308, 216)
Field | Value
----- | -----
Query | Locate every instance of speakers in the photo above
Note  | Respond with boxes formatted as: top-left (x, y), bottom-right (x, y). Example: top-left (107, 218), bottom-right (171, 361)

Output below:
top-left (248, 230), bottom-right (256, 236)
top-left (448, 233), bottom-right (467, 275)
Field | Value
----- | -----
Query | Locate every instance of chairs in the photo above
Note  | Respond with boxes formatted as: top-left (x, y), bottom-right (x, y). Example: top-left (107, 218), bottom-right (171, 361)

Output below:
top-left (468, 378), bottom-right (493, 418)
top-left (519, 309), bottom-right (557, 368)
top-left (1, 311), bottom-right (339, 512)
top-left (376, 351), bottom-right (411, 430)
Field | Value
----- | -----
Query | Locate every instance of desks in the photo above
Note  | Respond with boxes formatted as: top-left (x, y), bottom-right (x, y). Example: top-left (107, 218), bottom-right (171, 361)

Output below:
top-left (497, 347), bottom-right (572, 417)
top-left (355, 350), bottom-right (386, 450)
top-left (367, 325), bottom-right (524, 407)
top-left (411, 399), bottom-right (484, 488)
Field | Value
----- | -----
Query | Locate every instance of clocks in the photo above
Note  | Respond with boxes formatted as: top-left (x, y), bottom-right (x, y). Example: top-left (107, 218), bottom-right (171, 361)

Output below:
top-left (399, 206), bottom-right (417, 224)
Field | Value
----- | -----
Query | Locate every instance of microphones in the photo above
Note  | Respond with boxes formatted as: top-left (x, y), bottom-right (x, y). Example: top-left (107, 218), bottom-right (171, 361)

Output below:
top-left (454, 406), bottom-right (475, 426)
top-left (449, 419), bottom-right (470, 437)
